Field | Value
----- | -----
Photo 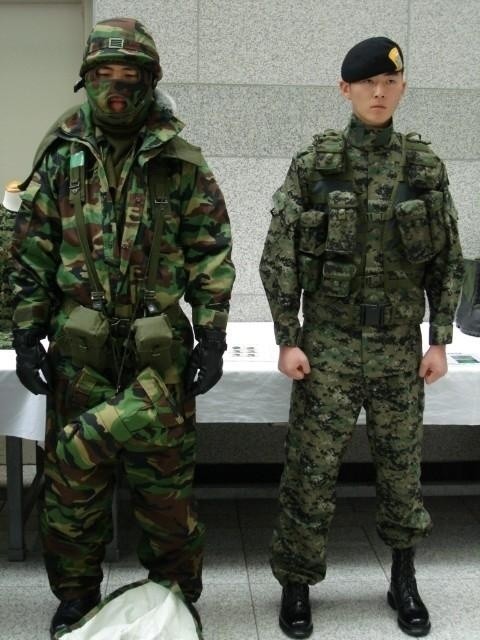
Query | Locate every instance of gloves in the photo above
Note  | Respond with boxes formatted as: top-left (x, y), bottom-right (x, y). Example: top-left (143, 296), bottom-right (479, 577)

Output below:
top-left (181, 324), bottom-right (226, 400)
top-left (11, 325), bottom-right (58, 396)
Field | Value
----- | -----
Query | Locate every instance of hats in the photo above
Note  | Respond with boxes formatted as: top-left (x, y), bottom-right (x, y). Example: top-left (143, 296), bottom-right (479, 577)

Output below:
top-left (341, 38), bottom-right (404, 82)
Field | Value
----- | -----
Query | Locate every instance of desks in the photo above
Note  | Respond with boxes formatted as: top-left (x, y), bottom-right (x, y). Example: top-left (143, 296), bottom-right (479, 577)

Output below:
top-left (2, 320), bottom-right (480, 563)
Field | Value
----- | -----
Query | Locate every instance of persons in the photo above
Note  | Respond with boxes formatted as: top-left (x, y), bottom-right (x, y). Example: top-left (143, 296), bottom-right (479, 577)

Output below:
top-left (0, 14), bottom-right (236, 639)
top-left (260, 37), bottom-right (469, 639)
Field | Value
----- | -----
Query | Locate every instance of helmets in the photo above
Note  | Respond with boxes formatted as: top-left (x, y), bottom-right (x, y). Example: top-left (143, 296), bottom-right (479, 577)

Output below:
top-left (80, 17), bottom-right (160, 78)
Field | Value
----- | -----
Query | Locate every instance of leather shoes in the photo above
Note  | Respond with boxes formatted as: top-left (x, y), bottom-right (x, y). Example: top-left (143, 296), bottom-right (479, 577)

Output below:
top-left (50, 585), bottom-right (101, 640)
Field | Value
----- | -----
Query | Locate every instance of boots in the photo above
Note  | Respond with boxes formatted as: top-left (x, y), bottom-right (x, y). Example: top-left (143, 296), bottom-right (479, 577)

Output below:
top-left (387, 544), bottom-right (431, 638)
top-left (278, 581), bottom-right (313, 639)
